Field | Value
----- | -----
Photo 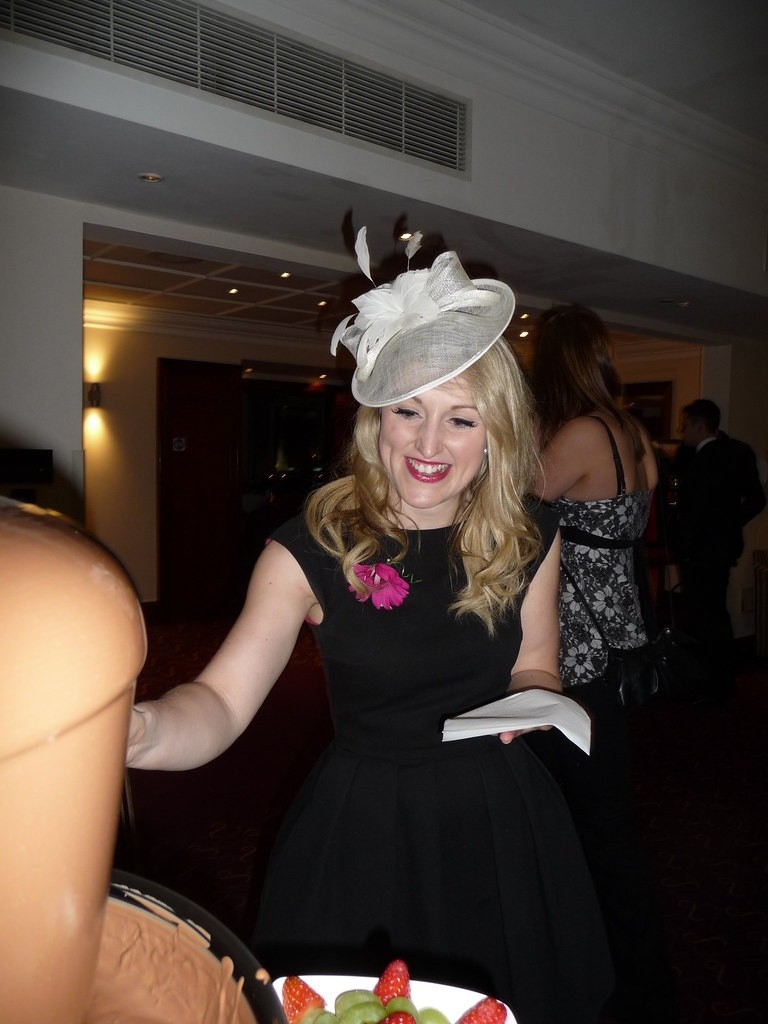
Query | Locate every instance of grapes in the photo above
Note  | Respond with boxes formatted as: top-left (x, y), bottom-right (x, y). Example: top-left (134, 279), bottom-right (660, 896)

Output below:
top-left (300, 989), bottom-right (450, 1024)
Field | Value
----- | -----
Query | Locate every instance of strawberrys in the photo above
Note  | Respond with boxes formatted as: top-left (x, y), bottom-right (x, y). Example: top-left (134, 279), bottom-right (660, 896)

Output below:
top-left (453, 998), bottom-right (507, 1024)
top-left (374, 960), bottom-right (410, 1007)
top-left (378, 1011), bottom-right (416, 1024)
top-left (282, 976), bottom-right (325, 1024)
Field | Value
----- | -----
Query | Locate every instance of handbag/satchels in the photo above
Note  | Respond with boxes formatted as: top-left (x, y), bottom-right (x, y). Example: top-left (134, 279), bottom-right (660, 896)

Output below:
top-left (607, 627), bottom-right (703, 711)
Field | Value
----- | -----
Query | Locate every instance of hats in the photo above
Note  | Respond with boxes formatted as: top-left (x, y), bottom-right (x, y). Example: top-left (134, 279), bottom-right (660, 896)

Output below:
top-left (330, 225), bottom-right (516, 407)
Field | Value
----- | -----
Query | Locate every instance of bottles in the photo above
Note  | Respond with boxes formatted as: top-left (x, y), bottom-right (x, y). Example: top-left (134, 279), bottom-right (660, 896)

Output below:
top-left (270, 444), bottom-right (288, 503)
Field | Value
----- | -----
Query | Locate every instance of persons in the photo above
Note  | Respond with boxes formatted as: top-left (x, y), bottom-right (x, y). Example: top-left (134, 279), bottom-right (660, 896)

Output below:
top-left (526, 305), bottom-right (658, 1024)
top-left (710, 403), bottom-right (766, 528)
top-left (126, 249), bottom-right (617, 1024)
top-left (659, 399), bottom-right (743, 703)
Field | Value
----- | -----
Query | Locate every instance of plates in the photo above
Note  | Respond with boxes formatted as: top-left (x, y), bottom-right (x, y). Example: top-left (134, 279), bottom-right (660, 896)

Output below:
top-left (274, 974), bottom-right (516, 1024)
